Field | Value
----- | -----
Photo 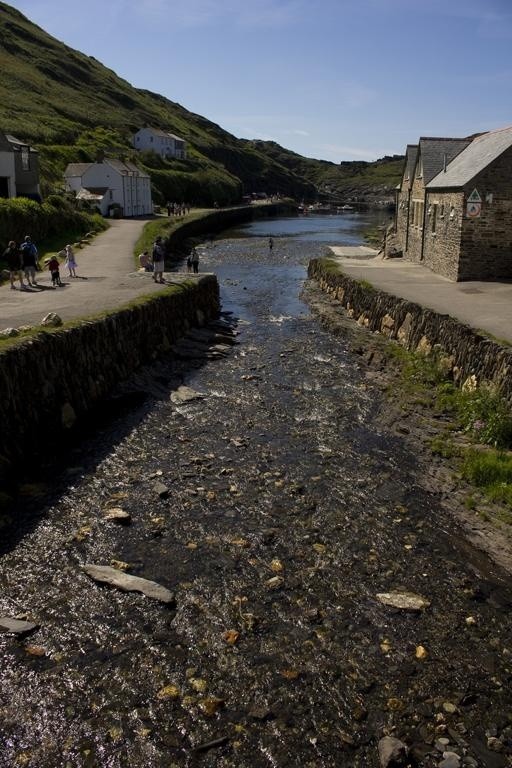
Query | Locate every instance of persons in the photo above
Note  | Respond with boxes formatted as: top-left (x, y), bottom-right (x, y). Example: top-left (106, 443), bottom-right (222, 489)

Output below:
top-left (153, 236), bottom-right (165, 284)
top-left (187, 256), bottom-right (193, 273)
top-left (166, 200), bottom-right (191, 217)
top-left (4, 241), bottom-right (29, 290)
top-left (139, 251), bottom-right (154, 272)
top-left (48, 256), bottom-right (62, 286)
top-left (191, 248), bottom-right (200, 273)
top-left (64, 244), bottom-right (79, 278)
top-left (268, 237), bottom-right (274, 252)
top-left (19, 235), bottom-right (39, 286)
